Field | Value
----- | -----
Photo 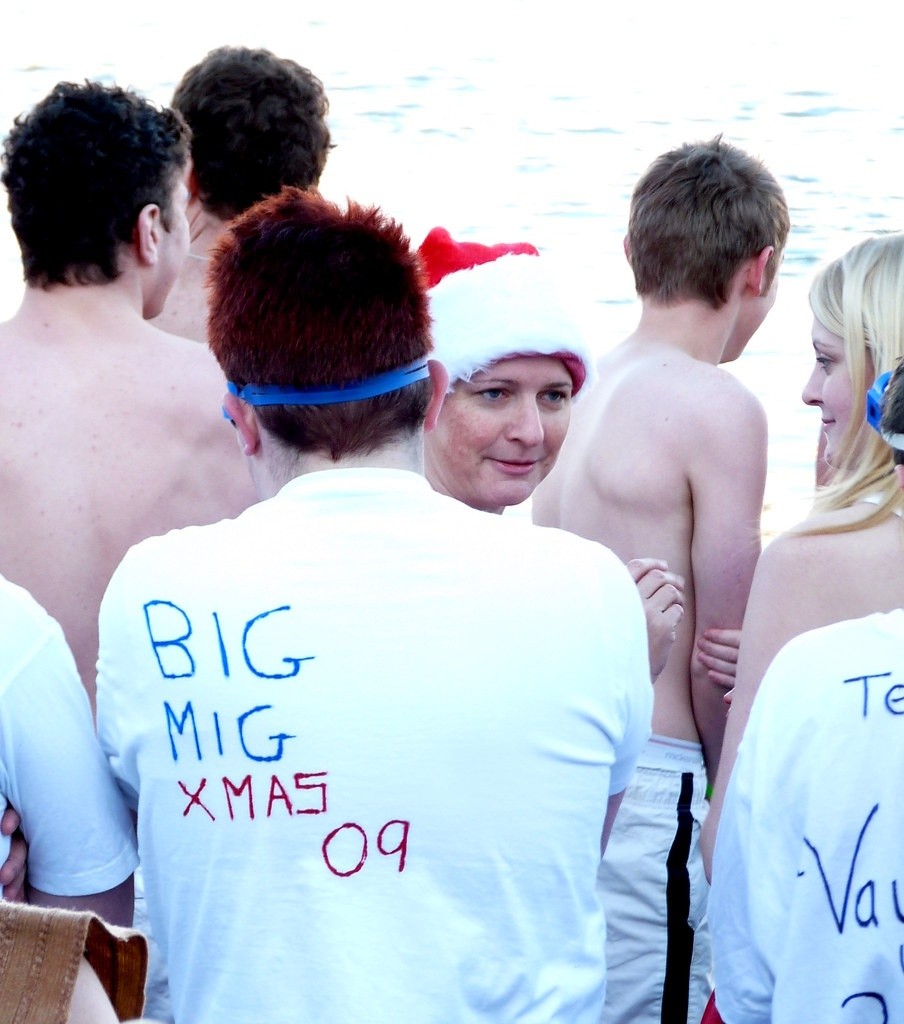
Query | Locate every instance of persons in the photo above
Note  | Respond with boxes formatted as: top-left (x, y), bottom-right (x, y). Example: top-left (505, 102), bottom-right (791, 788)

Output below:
top-left (705, 353), bottom-right (904, 1024)
top-left (697, 224), bottom-right (904, 889)
top-left (409, 227), bottom-right (686, 691)
top-left (92, 184), bottom-right (659, 1023)
top-left (533, 131), bottom-right (791, 1024)
top-left (0, 578), bottom-right (141, 1024)
top-left (139, 44), bottom-right (335, 344)
top-left (2, 78), bottom-right (265, 735)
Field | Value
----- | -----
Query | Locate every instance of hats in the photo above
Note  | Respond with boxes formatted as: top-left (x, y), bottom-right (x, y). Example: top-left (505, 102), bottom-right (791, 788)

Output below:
top-left (414, 227), bottom-right (588, 396)
